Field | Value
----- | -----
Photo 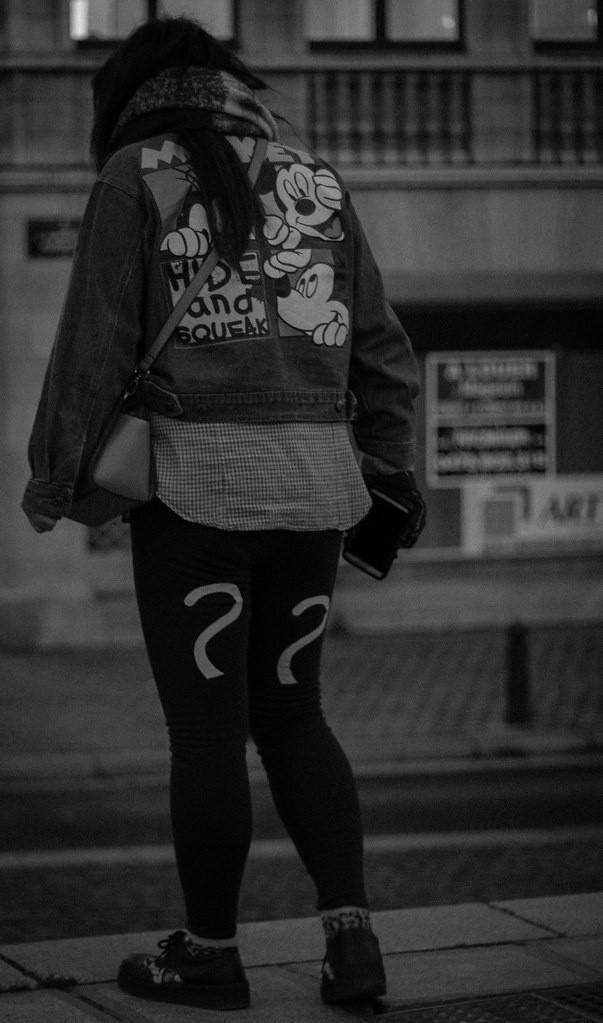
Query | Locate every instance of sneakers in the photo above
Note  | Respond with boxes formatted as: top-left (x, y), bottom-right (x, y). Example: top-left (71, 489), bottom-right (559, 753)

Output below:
top-left (319, 930), bottom-right (387, 1006)
top-left (117, 929), bottom-right (251, 1011)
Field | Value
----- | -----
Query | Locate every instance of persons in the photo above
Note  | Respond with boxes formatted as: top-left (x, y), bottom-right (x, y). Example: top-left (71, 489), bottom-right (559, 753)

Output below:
top-left (21, 13), bottom-right (426, 1011)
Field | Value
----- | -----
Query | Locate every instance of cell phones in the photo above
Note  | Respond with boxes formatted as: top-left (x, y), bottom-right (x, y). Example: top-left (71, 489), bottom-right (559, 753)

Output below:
top-left (342, 488), bottom-right (413, 580)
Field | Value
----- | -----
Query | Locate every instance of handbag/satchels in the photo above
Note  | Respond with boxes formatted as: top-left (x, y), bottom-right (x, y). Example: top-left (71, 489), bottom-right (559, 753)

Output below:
top-left (62, 389), bottom-right (159, 528)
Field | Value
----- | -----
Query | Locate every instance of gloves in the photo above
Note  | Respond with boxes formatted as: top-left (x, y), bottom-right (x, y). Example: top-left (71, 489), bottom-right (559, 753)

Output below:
top-left (363, 470), bottom-right (427, 559)
